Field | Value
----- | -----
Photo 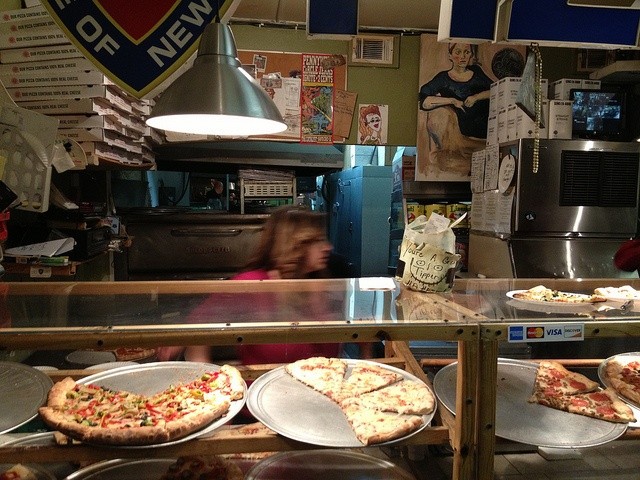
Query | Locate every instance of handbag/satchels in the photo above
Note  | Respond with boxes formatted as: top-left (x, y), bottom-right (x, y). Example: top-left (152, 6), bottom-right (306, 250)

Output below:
top-left (396, 199), bottom-right (467, 292)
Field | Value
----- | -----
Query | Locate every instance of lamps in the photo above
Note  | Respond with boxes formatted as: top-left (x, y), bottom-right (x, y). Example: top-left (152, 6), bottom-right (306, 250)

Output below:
top-left (144, 21), bottom-right (289, 139)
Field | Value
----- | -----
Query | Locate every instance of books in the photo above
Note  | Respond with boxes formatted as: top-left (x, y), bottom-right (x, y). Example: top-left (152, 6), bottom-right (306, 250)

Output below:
top-left (15, 255), bottom-right (70, 267)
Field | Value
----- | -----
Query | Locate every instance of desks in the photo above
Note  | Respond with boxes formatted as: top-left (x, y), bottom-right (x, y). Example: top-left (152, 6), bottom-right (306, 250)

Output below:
top-left (4, 236), bottom-right (136, 281)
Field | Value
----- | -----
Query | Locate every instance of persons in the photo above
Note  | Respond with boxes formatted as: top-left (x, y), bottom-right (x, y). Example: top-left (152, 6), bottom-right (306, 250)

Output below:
top-left (205, 180), bottom-right (225, 204)
top-left (361, 102), bottom-right (391, 148)
top-left (181, 204), bottom-right (346, 366)
top-left (614, 235), bottom-right (640, 278)
top-left (420, 36), bottom-right (501, 139)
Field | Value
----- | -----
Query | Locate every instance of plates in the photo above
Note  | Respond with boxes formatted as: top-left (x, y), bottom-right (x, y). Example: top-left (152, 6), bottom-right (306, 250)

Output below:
top-left (505, 288), bottom-right (592, 305)
top-left (506, 300), bottom-right (598, 314)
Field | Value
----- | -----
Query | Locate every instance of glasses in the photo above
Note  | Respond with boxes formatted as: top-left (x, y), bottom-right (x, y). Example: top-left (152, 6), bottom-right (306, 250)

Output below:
top-left (367, 119), bottom-right (380, 124)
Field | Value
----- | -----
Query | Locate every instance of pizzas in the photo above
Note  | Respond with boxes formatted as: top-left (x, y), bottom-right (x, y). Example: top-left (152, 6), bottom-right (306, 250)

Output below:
top-left (604, 361), bottom-right (640, 407)
top-left (512, 284), bottom-right (608, 308)
top-left (281, 354), bottom-right (438, 448)
top-left (527, 358), bottom-right (637, 425)
top-left (34, 361), bottom-right (247, 451)
top-left (0, 460), bottom-right (44, 480)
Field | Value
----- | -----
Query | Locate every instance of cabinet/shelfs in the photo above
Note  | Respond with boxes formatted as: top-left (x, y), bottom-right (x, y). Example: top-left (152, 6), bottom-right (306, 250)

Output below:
top-left (410, 277), bottom-right (640, 480)
top-left (327, 165), bottom-right (394, 277)
top-left (0, 276), bottom-right (481, 479)
